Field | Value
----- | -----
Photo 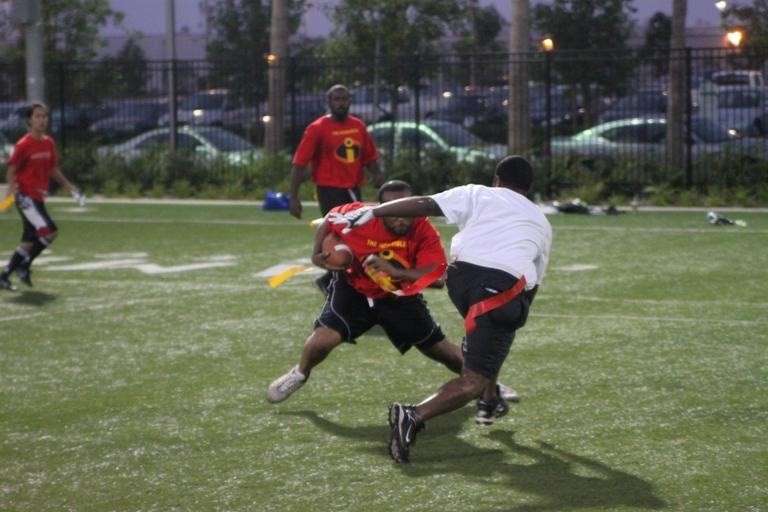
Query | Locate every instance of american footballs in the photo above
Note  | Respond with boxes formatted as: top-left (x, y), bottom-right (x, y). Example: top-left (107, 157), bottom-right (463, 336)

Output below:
top-left (321, 231), bottom-right (353, 268)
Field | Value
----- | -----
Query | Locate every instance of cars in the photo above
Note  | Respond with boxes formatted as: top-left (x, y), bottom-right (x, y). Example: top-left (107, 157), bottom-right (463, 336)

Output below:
top-left (541, 116), bottom-right (768, 184)
top-left (0, 96), bottom-right (99, 133)
top-left (363, 120), bottom-right (508, 184)
top-left (94, 125), bottom-right (262, 175)
top-left (1, 141), bottom-right (76, 188)
top-left (89, 91), bottom-right (252, 129)
top-left (326, 82), bottom-right (394, 123)
top-left (257, 93), bottom-right (323, 130)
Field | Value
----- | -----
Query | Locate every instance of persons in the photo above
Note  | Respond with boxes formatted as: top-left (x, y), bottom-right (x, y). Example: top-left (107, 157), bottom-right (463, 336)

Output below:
top-left (326, 155), bottom-right (553, 465)
top-left (289, 84), bottom-right (387, 304)
top-left (0, 102), bottom-right (85, 291)
top-left (262, 178), bottom-right (520, 404)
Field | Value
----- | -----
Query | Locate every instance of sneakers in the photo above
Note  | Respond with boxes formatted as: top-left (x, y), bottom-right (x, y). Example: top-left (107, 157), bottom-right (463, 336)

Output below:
top-left (474, 382), bottom-right (519, 427)
top-left (387, 401), bottom-right (426, 464)
top-left (267, 363), bottom-right (311, 404)
top-left (0, 267), bottom-right (33, 291)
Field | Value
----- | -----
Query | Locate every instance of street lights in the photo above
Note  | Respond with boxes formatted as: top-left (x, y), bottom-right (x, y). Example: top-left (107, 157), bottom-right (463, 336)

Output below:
top-left (541, 38), bottom-right (556, 200)
top-left (724, 28), bottom-right (746, 68)
